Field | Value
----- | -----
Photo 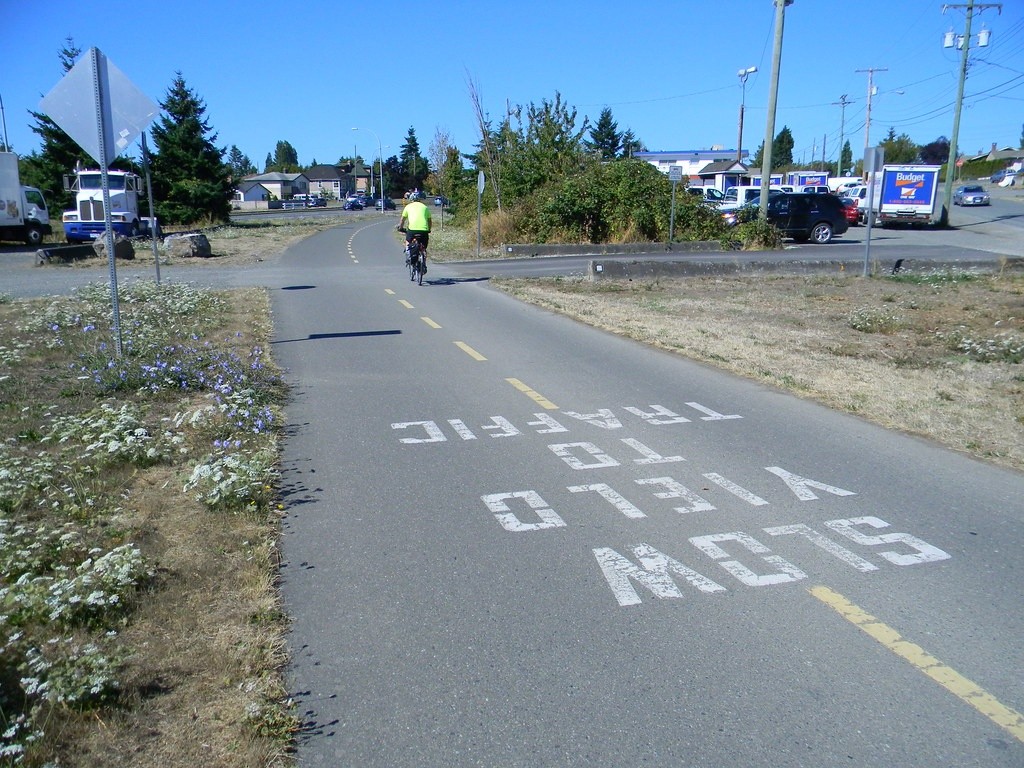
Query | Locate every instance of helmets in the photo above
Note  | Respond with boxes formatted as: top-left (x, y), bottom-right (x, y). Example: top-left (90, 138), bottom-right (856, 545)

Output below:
top-left (409, 191), bottom-right (420, 202)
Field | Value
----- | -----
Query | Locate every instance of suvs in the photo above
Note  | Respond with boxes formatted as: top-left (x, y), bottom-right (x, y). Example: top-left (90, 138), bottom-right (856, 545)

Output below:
top-left (719, 191), bottom-right (849, 244)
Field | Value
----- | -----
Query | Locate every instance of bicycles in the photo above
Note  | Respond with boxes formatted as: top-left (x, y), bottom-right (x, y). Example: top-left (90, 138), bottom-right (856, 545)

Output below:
top-left (396, 227), bottom-right (431, 286)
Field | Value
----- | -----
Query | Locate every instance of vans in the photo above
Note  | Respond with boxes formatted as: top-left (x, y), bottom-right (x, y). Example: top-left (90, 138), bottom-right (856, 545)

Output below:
top-left (292, 192), bottom-right (316, 203)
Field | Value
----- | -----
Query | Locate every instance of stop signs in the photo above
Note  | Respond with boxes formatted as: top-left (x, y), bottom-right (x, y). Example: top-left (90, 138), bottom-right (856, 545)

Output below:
top-left (957, 159), bottom-right (963, 167)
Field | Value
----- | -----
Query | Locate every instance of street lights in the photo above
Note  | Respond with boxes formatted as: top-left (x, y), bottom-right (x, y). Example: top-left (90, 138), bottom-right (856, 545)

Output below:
top-left (352, 127), bottom-right (385, 214)
top-left (370, 145), bottom-right (391, 200)
top-left (861, 86), bottom-right (905, 186)
top-left (736, 66), bottom-right (758, 163)
top-left (940, 29), bottom-right (993, 229)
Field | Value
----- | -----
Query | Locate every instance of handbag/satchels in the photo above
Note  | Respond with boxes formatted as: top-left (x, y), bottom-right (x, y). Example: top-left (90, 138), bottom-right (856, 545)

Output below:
top-left (406, 243), bottom-right (418, 264)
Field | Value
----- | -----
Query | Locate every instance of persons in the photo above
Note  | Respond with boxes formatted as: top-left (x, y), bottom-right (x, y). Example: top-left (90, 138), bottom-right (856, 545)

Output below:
top-left (398, 195), bottom-right (432, 274)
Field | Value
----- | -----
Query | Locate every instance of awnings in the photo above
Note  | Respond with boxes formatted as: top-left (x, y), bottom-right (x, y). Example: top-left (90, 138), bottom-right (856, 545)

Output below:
top-left (987, 150), bottom-right (1024, 161)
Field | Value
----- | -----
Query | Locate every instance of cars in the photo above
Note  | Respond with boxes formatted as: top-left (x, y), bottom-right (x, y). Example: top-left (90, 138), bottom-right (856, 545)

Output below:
top-left (344, 190), bottom-right (397, 210)
top-left (308, 197), bottom-right (329, 207)
top-left (991, 169), bottom-right (1019, 184)
top-left (435, 197), bottom-right (451, 208)
top-left (952, 184), bottom-right (991, 207)
top-left (403, 189), bottom-right (426, 202)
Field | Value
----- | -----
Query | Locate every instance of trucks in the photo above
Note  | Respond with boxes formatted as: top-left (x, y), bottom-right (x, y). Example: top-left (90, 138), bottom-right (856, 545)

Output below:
top-left (0, 151), bottom-right (53, 249)
top-left (685, 164), bottom-right (942, 227)
top-left (61, 160), bottom-right (163, 245)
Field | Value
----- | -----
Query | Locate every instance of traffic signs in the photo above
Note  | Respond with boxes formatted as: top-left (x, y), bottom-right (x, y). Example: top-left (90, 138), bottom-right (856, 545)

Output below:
top-left (668, 165), bottom-right (682, 181)
